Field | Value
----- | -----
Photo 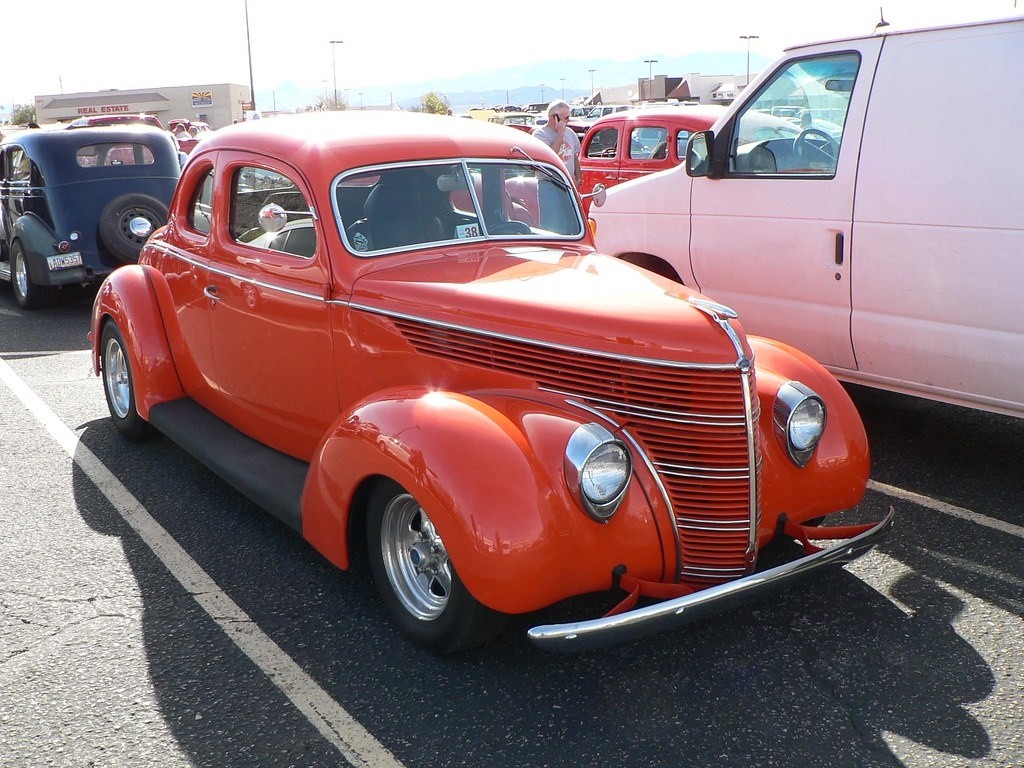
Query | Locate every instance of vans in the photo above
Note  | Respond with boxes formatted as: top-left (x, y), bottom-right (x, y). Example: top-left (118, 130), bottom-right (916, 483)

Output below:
top-left (578, 111), bottom-right (805, 215)
top-left (587, 10), bottom-right (1024, 423)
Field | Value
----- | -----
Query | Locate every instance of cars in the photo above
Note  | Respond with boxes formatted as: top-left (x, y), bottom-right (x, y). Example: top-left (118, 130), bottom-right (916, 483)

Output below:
top-left (1, 115), bottom-right (215, 309)
top-left (471, 98), bottom-right (699, 157)
top-left (87, 110), bottom-right (897, 646)
top-left (759, 106), bottom-right (843, 143)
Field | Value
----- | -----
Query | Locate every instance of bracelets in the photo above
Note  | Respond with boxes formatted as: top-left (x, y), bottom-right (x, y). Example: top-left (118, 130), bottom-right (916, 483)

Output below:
top-left (577, 179), bottom-right (582, 185)
top-left (575, 174), bottom-right (581, 177)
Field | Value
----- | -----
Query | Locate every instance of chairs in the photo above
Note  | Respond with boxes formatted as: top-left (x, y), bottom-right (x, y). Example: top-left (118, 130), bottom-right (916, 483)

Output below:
top-left (237, 170), bottom-right (447, 257)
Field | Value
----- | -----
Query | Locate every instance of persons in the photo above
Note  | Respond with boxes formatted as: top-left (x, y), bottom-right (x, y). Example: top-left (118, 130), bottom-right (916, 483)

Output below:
top-left (152, 130), bottom-right (188, 173)
top-left (532, 99), bottom-right (583, 236)
top-left (446, 108), bottom-right (454, 117)
top-left (234, 120), bottom-right (237, 125)
top-left (172, 124), bottom-right (200, 138)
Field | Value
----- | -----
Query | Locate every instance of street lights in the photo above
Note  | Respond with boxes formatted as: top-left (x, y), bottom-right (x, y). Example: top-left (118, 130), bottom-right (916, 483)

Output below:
top-left (643, 59), bottom-right (658, 98)
top-left (588, 69), bottom-right (597, 104)
top-left (540, 83), bottom-right (545, 103)
top-left (739, 35), bottom-right (759, 83)
top-left (560, 78), bottom-right (567, 99)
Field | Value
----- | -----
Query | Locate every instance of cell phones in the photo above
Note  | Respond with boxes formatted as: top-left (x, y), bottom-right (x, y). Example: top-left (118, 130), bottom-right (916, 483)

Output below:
top-left (553, 114), bottom-right (560, 123)
